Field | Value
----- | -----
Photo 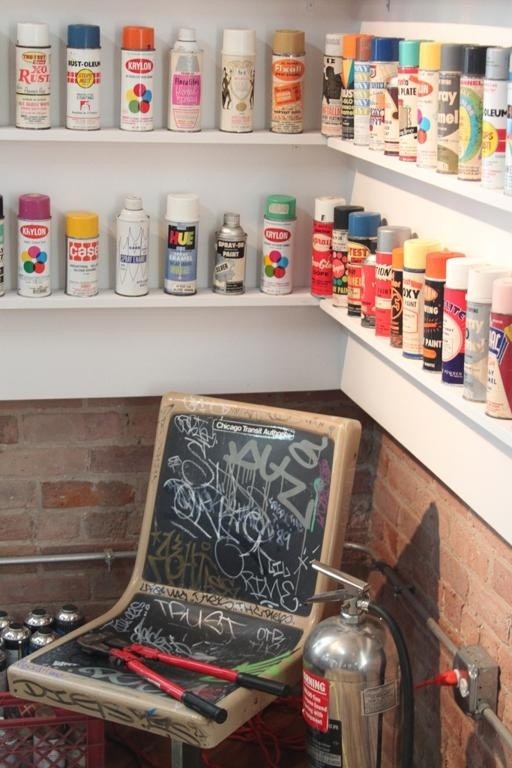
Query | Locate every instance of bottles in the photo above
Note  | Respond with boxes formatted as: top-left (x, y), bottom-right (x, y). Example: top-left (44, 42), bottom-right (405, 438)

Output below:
top-left (211, 212), bottom-right (247, 296)
top-left (309, 198), bottom-right (512, 421)
top-left (113, 195), bottom-right (150, 297)
top-left (318, 31), bottom-right (512, 198)
top-left (119, 23), bottom-right (158, 133)
top-left (62, 24), bottom-right (105, 130)
top-left (269, 28), bottom-right (306, 134)
top-left (161, 193), bottom-right (200, 296)
top-left (0, 604), bottom-right (86, 692)
top-left (219, 27), bottom-right (256, 135)
top-left (15, 23), bottom-right (53, 131)
top-left (15, 193), bottom-right (52, 298)
top-left (167, 27), bottom-right (205, 134)
top-left (258, 193), bottom-right (297, 296)
top-left (64, 211), bottom-right (101, 296)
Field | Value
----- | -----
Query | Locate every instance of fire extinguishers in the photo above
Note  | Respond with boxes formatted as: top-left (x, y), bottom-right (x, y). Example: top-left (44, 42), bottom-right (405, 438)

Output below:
top-left (302, 559), bottom-right (413, 768)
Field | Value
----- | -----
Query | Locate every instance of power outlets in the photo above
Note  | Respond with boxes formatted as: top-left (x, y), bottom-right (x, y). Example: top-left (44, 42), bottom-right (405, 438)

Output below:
top-left (451, 646), bottom-right (500, 720)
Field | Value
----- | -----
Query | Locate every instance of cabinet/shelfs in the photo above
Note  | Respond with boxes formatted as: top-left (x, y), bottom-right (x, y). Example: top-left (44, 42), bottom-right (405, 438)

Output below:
top-left (1, 123), bottom-right (512, 459)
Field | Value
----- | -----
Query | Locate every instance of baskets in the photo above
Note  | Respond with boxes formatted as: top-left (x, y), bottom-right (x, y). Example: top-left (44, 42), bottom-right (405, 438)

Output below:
top-left (0, 690), bottom-right (106, 768)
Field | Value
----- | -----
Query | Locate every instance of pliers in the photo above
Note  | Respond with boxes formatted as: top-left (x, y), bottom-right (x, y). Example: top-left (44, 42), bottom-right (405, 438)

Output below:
top-left (78, 631), bottom-right (288, 724)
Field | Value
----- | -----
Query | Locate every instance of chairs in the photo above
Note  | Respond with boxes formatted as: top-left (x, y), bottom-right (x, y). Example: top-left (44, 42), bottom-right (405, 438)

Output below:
top-left (0, 388), bottom-right (363, 766)
